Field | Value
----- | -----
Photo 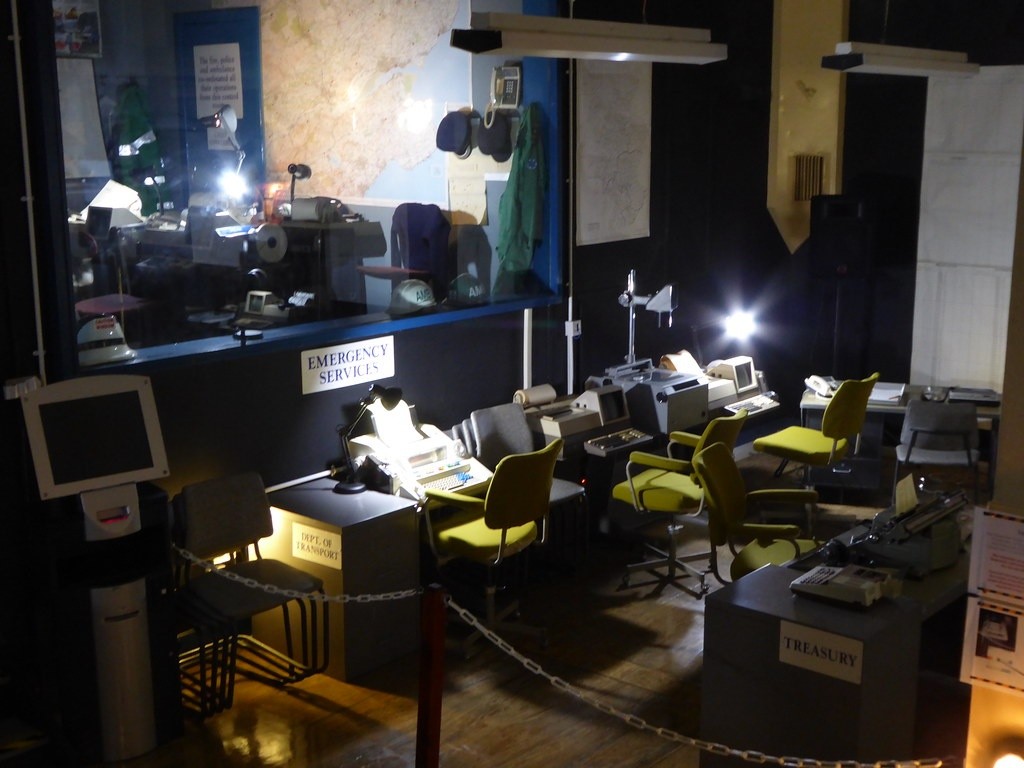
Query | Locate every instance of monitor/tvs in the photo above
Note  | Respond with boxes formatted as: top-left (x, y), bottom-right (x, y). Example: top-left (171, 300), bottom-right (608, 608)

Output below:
top-left (85, 205), bottom-right (145, 241)
top-left (19, 375), bottom-right (172, 501)
top-left (706, 356), bottom-right (759, 394)
top-left (568, 384), bottom-right (630, 428)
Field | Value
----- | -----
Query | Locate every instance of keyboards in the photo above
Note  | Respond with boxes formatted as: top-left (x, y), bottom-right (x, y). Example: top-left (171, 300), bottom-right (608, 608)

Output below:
top-left (724, 395), bottom-right (780, 418)
top-left (584, 427), bottom-right (653, 456)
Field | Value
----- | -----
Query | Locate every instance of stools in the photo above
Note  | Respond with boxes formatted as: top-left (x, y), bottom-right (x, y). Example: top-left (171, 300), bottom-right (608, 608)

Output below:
top-left (74, 293), bottom-right (153, 348)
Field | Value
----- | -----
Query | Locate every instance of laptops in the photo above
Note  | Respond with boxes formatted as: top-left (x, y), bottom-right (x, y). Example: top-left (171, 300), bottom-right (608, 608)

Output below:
top-left (948, 387), bottom-right (1001, 408)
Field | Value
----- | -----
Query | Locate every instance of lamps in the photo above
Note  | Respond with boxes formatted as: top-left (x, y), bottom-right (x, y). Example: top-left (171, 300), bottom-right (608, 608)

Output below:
top-left (246, 267), bottom-right (295, 312)
top-left (820, 0), bottom-right (981, 81)
top-left (287, 163), bottom-right (312, 204)
top-left (450, 0), bottom-right (728, 68)
top-left (132, 164), bottom-right (165, 217)
top-left (332, 383), bottom-right (403, 495)
top-left (198, 105), bottom-right (247, 175)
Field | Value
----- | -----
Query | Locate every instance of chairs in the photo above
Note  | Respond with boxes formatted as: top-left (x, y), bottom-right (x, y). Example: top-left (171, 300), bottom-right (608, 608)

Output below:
top-left (694, 440), bottom-right (827, 587)
top-left (890, 397), bottom-right (983, 506)
top-left (452, 402), bottom-right (589, 592)
top-left (166, 468), bottom-right (330, 723)
top-left (611, 412), bottom-right (752, 594)
top-left (753, 373), bottom-right (881, 524)
top-left (419, 439), bottom-right (567, 663)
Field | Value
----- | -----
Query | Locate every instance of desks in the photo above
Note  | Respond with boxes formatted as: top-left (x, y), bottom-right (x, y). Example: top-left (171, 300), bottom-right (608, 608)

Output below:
top-left (249, 478), bottom-right (423, 683)
top-left (781, 524), bottom-right (977, 679)
top-left (799, 380), bottom-right (1002, 486)
top-left (697, 542), bottom-right (971, 768)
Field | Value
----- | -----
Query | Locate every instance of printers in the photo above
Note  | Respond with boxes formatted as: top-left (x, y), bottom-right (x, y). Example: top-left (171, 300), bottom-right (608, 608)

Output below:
top-left (698, 490), bottom-right (981, 767)
top-left (349, 403), bottom-right (495, 516)
top-left (605, 358), bottom-right (708, 433)
